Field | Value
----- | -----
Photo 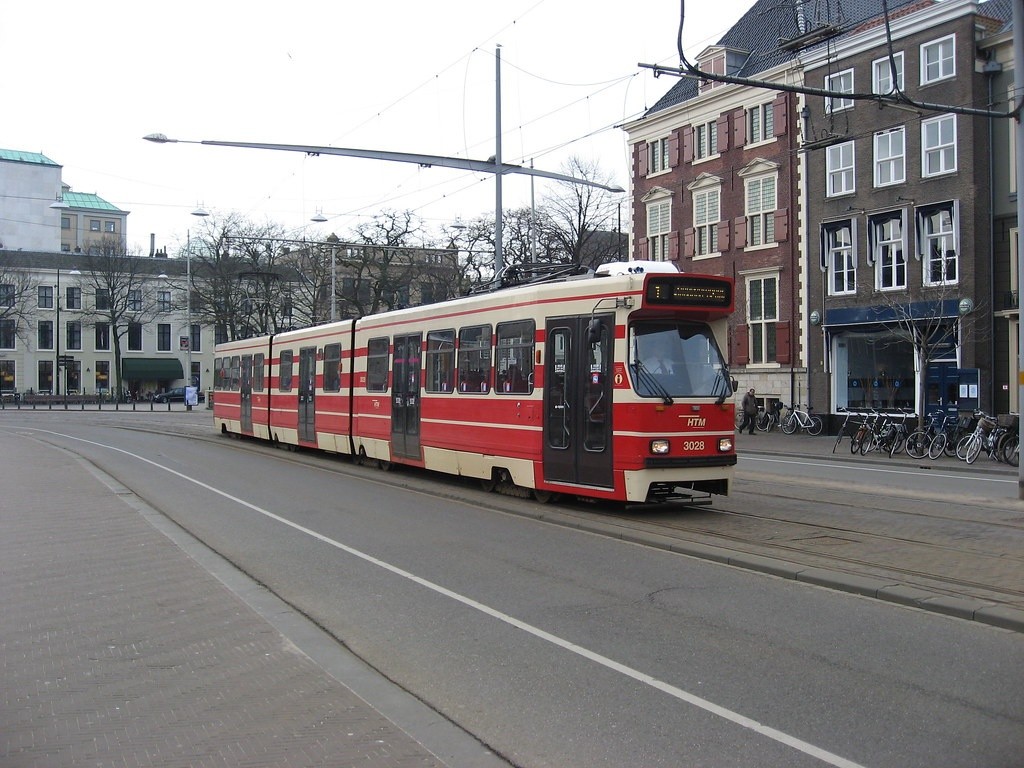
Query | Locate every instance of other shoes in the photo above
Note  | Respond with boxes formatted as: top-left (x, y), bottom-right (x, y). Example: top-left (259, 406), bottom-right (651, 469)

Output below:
top-left (749, 432), bottom-right (756, 435)
top-left (739, 426), bottom-right (742, 433)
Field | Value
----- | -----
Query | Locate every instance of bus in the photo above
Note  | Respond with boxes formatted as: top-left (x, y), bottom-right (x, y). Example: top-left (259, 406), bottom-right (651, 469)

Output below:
top-left (213, 260), bottom-right (738, 508)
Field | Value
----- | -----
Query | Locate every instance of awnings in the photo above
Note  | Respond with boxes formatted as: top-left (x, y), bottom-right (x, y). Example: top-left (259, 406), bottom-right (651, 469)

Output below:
top-left (123, 358), bottom-right (184, 379)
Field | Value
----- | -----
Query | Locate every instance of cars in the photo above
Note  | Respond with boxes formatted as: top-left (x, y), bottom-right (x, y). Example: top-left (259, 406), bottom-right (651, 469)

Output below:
top-left (154, 387), bottom-right (205, 404)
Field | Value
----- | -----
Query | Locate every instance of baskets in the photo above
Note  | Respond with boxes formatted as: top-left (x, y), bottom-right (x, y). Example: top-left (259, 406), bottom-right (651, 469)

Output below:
top-left (964, 417), bottom-right (977, 433)
top-left (978, 418), bottom-right (996, 434)
top-left (997, 414), bottom-right (1016, 428)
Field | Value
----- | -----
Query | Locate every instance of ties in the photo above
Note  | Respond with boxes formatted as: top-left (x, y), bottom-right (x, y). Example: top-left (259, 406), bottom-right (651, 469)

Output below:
top-left (658, 360), bottom-right (668, 375)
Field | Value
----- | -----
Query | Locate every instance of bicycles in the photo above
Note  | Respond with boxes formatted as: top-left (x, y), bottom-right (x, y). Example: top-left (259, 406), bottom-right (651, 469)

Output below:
top-left (832, 406), bottom-right (1019, 466)
top-left (734, 401), bottom-right (783, 432)
top-left (781, 403), bottom-right (823, 436)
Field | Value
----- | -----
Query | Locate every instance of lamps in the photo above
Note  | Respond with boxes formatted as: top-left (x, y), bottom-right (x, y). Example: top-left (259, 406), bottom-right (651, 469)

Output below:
top-left (959, 298), bottom-right (974, 315)
top-left (810, 308), bottom-right (822, 327)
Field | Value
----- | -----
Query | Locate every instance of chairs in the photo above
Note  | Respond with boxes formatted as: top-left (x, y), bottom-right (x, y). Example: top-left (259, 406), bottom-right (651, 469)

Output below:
top-left (442, 381), bottom-right (511, 392)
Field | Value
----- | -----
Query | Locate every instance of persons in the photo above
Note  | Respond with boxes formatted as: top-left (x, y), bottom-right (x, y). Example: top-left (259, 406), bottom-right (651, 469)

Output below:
top-left (334, 370), bottom-right (340, 390)
top-left (464, 371), bottom-right (474, 392)
top-left (130, 387), bottom-right (137, 401)
top-left (739, 389), bottom-right (759, 435)
top-left (505, 366), bottom-right (527, 392)
top-left (552, 373), bottom-right (562, 445)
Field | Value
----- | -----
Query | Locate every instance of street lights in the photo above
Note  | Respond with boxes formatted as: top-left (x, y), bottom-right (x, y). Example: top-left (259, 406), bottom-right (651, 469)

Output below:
top-left (143, 132), bottom-right (626, 292)
